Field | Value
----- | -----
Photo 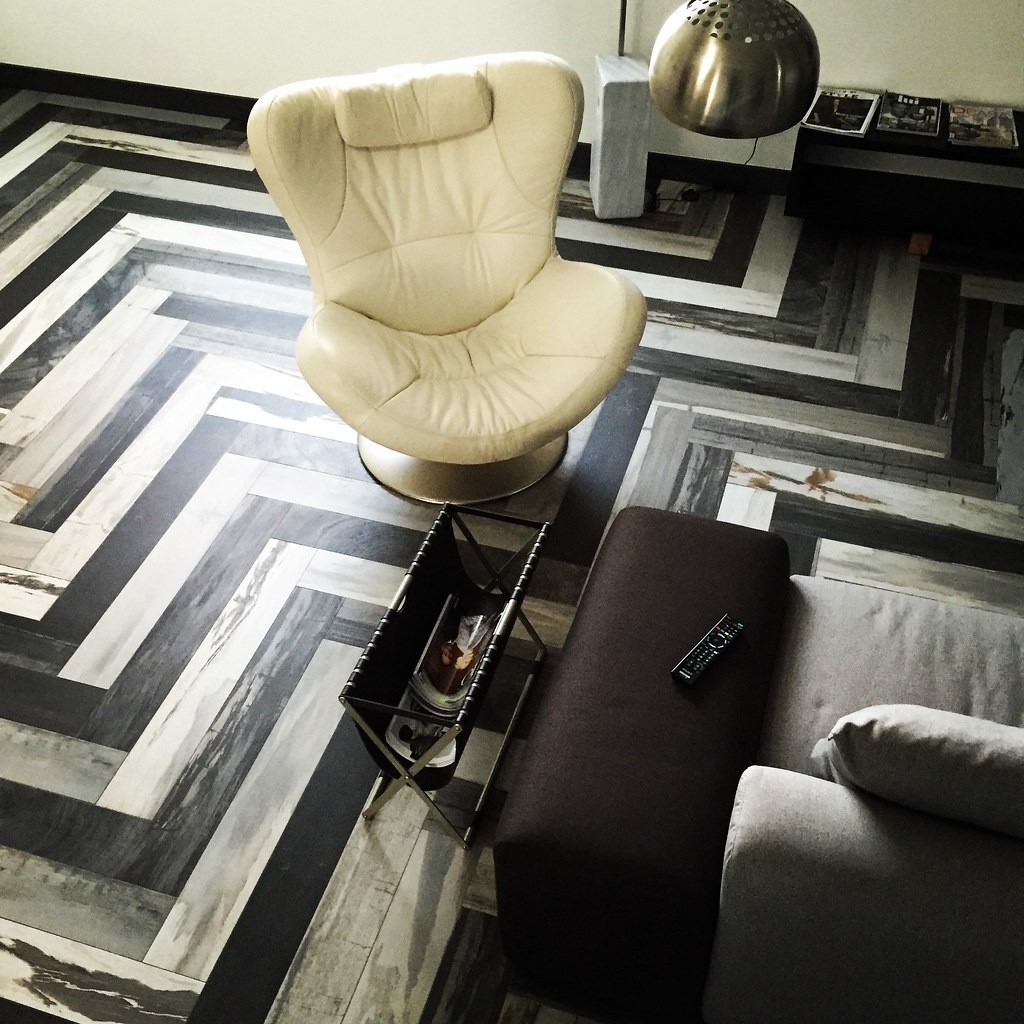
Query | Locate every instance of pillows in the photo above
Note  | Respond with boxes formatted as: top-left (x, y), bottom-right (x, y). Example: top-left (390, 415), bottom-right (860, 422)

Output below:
top-left (811, 702), bottom-right (1024, 841)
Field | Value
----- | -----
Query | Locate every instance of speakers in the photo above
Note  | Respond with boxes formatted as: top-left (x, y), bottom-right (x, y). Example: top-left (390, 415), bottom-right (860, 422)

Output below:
top-left (587, 52), bottom-right (651, 217)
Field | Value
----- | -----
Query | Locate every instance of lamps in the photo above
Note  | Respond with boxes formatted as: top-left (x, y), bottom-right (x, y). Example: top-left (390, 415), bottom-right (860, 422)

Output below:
top-left (648, 0), bottom-right (820, 141)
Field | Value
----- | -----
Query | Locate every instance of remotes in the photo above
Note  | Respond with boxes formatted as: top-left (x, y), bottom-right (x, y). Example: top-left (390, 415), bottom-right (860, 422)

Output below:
top-left (670, 612), bottom-right (746, 686)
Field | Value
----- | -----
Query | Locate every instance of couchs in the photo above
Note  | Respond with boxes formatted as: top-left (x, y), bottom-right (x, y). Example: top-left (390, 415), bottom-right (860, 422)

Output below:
top-left (707, 507), bottom-right (1024, 1024)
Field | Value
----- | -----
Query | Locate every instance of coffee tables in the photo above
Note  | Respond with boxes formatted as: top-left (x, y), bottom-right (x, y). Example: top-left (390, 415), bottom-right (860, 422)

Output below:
top-left (483, 502), bottom-right (791, 1024)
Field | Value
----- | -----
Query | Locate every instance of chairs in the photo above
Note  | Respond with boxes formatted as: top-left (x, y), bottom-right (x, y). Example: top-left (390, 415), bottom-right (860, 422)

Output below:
top-left (245, 49), bottom-right (649, 507)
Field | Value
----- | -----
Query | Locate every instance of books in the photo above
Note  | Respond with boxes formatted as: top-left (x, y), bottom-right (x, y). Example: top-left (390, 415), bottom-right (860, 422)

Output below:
top-left (382, 581), bottom-right (510, 769)
top-left (800, 88), bottom-right (882, 139)
top-left (876, 89), bottom-right (943, 137)
top-left (947, 103), bottom-right (1020, 150)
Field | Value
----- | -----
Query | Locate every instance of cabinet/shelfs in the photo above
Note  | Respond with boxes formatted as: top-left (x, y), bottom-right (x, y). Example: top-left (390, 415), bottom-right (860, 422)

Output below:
top-left (337, 500), bottom-right (558, 853)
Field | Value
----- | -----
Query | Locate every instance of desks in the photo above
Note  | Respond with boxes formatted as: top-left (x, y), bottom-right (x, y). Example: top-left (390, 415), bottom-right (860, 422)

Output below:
top-left (783, 87), bottom-right (1024, 252)
